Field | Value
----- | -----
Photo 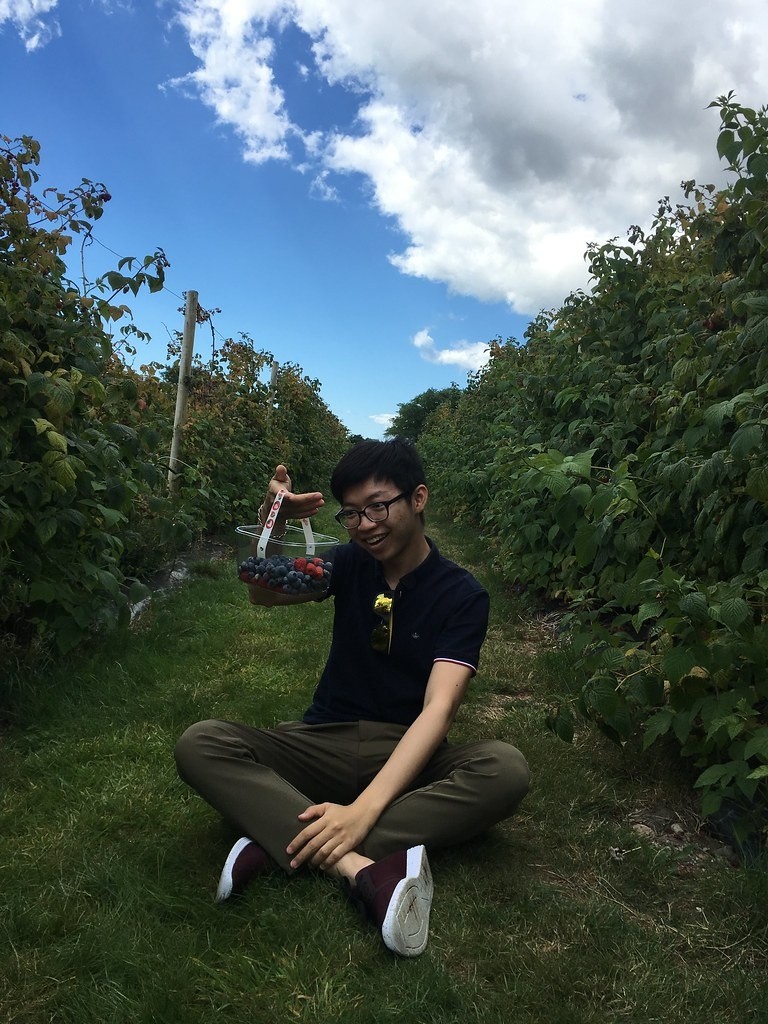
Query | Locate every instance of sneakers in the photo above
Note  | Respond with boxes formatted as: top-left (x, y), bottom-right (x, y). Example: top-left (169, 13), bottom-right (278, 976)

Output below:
top-left (355, 845), bottom-right (434, 959)
top-left (214, 836), bottom-right (269, 906)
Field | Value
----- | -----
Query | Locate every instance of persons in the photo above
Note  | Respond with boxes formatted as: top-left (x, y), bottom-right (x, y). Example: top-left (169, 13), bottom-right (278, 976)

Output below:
top-left (174, 437), bottom-right (531, 958)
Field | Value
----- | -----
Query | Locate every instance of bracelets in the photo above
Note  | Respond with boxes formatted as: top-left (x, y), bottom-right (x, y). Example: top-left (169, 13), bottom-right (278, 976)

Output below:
top-left (257, 503), bottom-right (288, 538)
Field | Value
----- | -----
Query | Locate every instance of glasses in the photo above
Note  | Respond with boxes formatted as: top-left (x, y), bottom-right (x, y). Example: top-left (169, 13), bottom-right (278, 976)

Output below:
top-left (335, 490), bottom-right (409, 530)
top-left (369, 591), bottom-right (394, 657)
